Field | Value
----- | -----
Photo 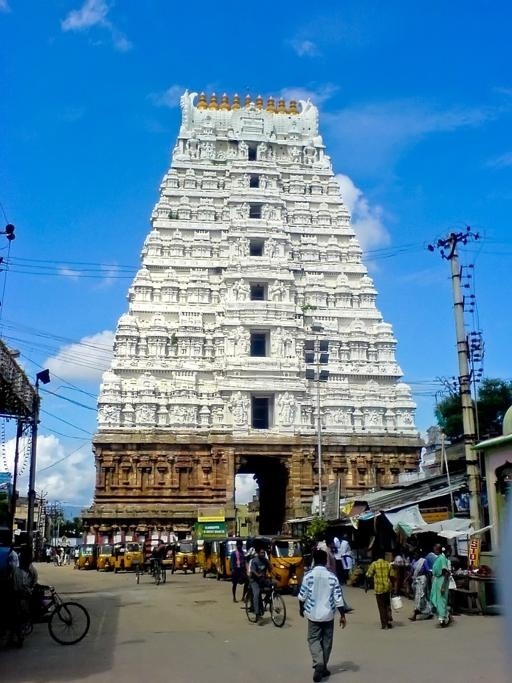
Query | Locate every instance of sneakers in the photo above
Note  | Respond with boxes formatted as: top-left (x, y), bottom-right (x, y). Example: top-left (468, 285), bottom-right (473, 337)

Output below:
top-left (313, 666), bottom-right (331, 682)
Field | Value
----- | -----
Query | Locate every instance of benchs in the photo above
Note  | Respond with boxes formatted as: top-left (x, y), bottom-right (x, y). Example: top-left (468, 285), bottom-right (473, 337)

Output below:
top-left (449, 589), bottom-right (484, 614)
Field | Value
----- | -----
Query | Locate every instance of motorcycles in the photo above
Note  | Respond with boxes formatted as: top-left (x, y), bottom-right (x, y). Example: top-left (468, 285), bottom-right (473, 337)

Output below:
top-left (160, 537), bottom-right (306, 596)
top-left (76, 538), bottom-right (166, 584)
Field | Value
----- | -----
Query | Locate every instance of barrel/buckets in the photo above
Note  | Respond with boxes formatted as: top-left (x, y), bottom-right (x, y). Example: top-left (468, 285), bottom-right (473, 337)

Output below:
top-left (391, 596), bottom-right (403, 609)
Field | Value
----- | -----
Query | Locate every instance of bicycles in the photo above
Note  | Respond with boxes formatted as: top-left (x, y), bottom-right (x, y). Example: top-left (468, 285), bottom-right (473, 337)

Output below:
top-left (22, 584), bottom-right (91, 645)
top-left (240, 580), bottom-right (286, 628)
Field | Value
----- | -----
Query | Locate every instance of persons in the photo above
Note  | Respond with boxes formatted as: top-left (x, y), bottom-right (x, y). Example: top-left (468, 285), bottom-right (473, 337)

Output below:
top-left (259, 140), bottom-right (269, 159)
top-left (13, 548), bottom-right (50, 623)
top-left (232, 277), bottom-right (251, 301)
top-left (237, 234), bottom-right (249, 256)
top-left (260, 173), bottom-right (270, 190)
top-left (401, 539), bottom-right (455, 627)
top-left (249, 546), bottom-right (277, 623)
top-left (268, 279), bottom-right (286, 300)
top-left (229, 538), bottom-right (247, 602)
top-left (278, 390), bottom-right (297, 426)
top-left (302, 139), bottom-right (319, 165)
top-left (45, 540), bottom-right (82, 568)
top-left (184, 130), bottom-right (202, 160)
top-left (266, 236), bottom-right (279, 259)
top-left (263, 202), bottom-right (272, 219)
top-left (230, 389), bottom-right (250, 426)
top-left (232, 324), bottom-right (252, 357)
top-left (239, 140), bottom-right (249, 156)
top-left (279, 328), bottom-right (297, 359)
top-left (241, 171), bottom-right (249, 189)
top-left (309, 532), bottom-right (357, 584)
top-left (239, 201), bottom-right (250, 220)
top-left (363, 547), bottom-right (396, 629)
top-left (296, 549), bottom-right (348, 682)
